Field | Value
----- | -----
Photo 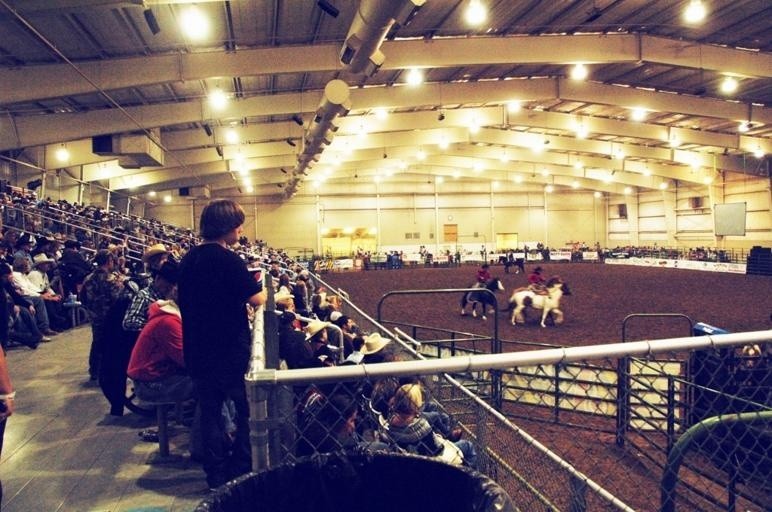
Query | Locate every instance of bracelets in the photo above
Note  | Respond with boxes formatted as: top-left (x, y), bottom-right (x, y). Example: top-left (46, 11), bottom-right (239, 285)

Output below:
top-left (1, 393), bottom-right (17, 404)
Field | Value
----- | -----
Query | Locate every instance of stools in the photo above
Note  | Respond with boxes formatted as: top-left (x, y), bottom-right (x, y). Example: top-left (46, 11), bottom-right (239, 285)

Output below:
top-left (131, 399), bottom-right (191, 465)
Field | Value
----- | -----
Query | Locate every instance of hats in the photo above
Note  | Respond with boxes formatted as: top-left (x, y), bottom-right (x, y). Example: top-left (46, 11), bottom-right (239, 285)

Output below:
top-left (280, 310), bottom-right (296, 324)
top-left (359, 332), bottom-right (391, 354)
top-left (17, 234), bottom-right (34, 247)
top-left (30, 254), bottom-right (55, 266)
top-left (36, 236), bottom-right (48, 246)
top-left (481, 264), bottom-right (489, 269)
top-left (142, 244), bottom-right (166, 261)
top-left (46, 235), bottom-right (56, 241)
top-left (108, 243), bottom-right (116, 250)
top-left (64, 239), bottom-right (81, 250)
top-left (303, 320), bottom-right (331, 341)
top-left (331, 311), bottom-right (343, 322)
top-left (534, 267), bottom-right (545, 273)
top-left (147, 261), bottom-right (180, 283)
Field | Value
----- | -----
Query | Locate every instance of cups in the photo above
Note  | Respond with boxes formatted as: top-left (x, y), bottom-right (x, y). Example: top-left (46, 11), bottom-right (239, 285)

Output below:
top-left (249, 270), bottom-right (262, 292)
top-left (69, 295), bottom-right (77, 304)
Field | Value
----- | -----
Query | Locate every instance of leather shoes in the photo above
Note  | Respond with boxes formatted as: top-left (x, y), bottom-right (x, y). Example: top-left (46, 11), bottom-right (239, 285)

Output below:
top-left (46, 329), bottom-right (57, 335)
top-left (42, 336), bottom-right (50, 341)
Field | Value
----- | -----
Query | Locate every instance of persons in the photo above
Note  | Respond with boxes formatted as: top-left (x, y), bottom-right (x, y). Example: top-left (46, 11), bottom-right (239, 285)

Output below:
top-left (79, 244), bottom-right (170, 384)
top-left (350, 246), bottom-right (462, 271)
top-left (473, 240), bottom-right (601, 279)
top-left (295, 273), bottom-right (477, 472)
top-left (1, 187), bottom-right (199, 260)
top-left (179, 200), bottom-right (295, 490)
top-left (1, 346), bottom-right (16, 452)
top-left (1, 228), bottom-right (98, 354)
top-left (298, 252), bottom-right (334, 273)
top-left (604, 243), bottom-right (730, 263)
top-left (127, 282), bottom-right (198, 431)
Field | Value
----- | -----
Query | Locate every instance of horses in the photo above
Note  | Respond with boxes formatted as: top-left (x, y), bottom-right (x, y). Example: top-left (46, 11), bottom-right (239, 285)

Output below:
top-left (499, 256), bottom-right (524, 274)
top-left (461, 277), bottom-right (505, 320)
top-left (511, 274), bottom-right (563, 326)
top-left (508, 282), bottom-right (571, 328)
